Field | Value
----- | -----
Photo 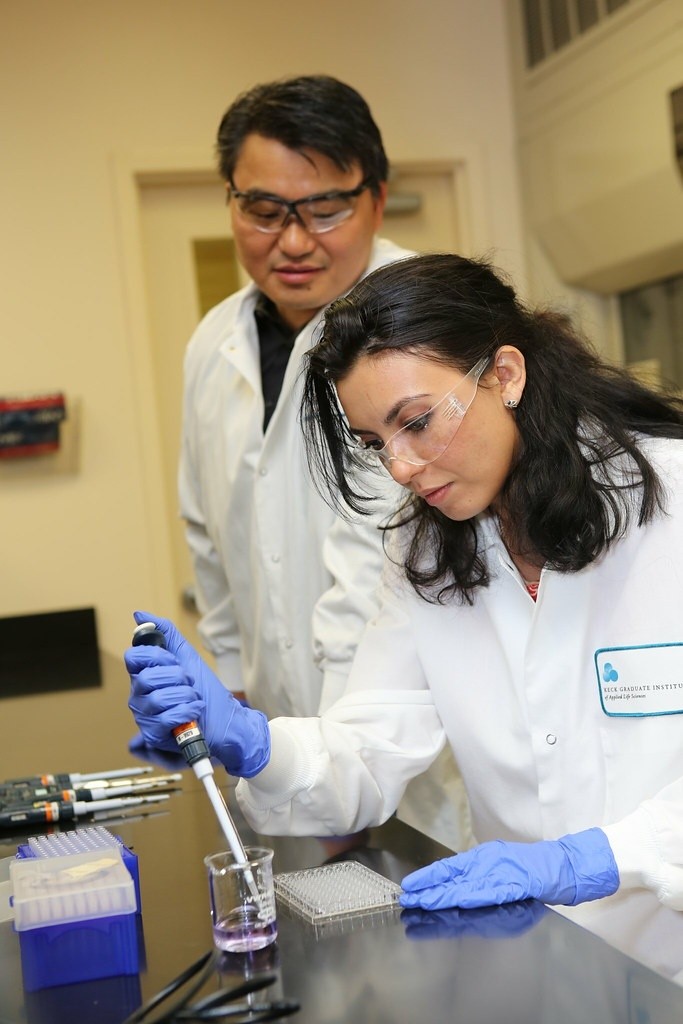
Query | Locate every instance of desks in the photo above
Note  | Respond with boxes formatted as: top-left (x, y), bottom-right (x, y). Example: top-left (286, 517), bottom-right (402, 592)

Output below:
top-left (1, 643), bottom-right (683, 1024)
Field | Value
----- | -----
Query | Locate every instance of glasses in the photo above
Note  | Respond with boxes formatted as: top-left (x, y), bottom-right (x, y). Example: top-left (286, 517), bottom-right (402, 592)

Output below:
top-left (352, 349), bottom-right (492, 481)
top-left (228, 178), bottom-right (369, 235)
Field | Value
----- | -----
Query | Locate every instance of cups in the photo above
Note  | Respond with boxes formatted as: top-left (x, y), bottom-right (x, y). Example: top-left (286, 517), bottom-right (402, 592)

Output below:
top-left (203, 847), bottom-right (279, 953)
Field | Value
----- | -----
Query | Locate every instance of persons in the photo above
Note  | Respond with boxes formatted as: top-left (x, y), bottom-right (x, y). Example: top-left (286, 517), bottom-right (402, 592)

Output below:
top-left (177, 73), bottom-right (481, 861)
top-left (124, 252), bottom-right (683, 984)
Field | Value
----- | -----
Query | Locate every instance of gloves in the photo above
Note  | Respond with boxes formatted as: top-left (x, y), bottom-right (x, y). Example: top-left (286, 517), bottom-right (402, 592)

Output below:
top-left (400, 898), bottom-right (548, 941)
top-left (398, 826), bottom-right (620, 911)
top-left (124, 610), bottom-right (272, 779)
